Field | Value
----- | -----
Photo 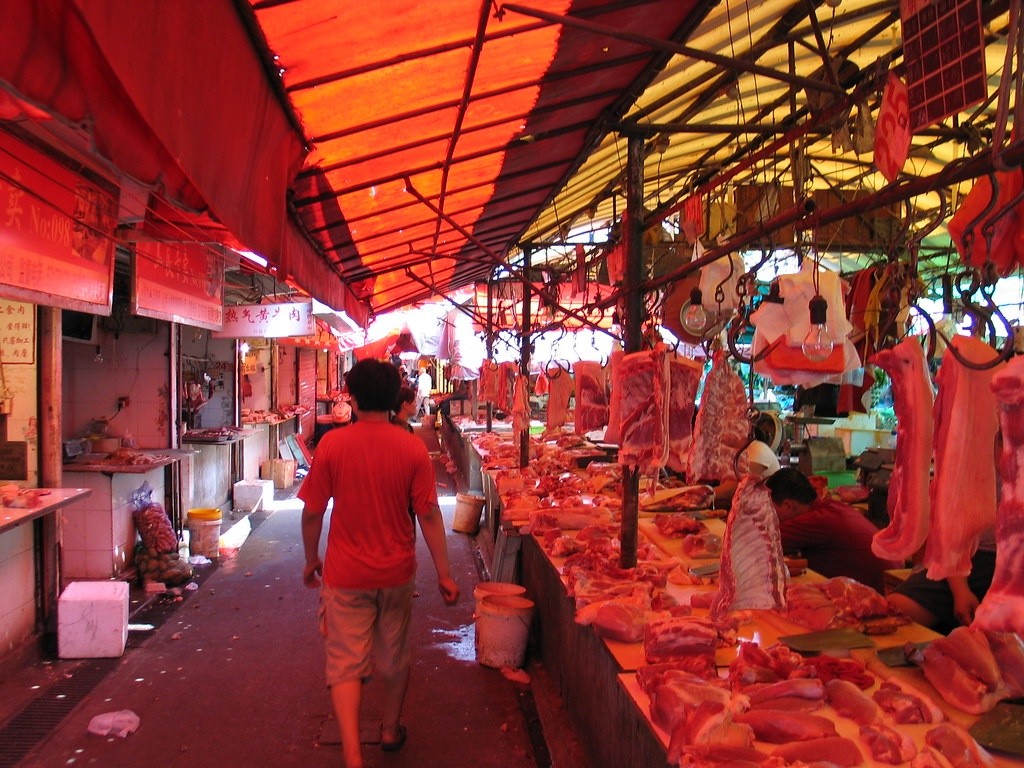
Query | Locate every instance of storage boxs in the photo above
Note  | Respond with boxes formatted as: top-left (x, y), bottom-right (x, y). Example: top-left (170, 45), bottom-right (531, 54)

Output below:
top-left (259, 458), bottom-right (294, 489)
top-left (57, 581), bottom-right (130, 659)
top-left (232, 478), bottom-right (274, 512)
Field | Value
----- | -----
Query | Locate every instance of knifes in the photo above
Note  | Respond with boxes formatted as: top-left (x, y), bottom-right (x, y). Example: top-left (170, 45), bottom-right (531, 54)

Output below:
top-left (684, 509), bottom-right (727, 520)
top-left (777, 624), bottom-right (897, 652)
top-left (874, 641), bottom-right (932, 668)
top-left (689, 563), bottom-right (719, 575)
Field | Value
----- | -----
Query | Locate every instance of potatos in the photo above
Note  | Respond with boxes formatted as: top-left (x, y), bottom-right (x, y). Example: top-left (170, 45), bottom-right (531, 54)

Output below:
top-left (133, 548), bottom-right (193, 586)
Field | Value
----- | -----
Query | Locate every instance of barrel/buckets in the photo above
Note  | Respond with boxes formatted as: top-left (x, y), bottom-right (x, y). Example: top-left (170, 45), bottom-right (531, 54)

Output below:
top-left (187, 507), bottom-right (222, 559)
top-left (452, 492), bottom-right (486, 535)
top-left (472, 582), bottom-right (526, 650)
top-left (425, 414), bottom-right (436, 429)
top-left (477, 594), bottom-right (534, 669)
top-left (316, 414), bottom-right (333, 442)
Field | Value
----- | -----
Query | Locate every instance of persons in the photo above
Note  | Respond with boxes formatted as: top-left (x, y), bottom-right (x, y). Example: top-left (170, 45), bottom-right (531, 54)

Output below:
top-left (390, 386), bottom-right (421, 598)
top-left (399, 366), bottom-right (432, 422)
top-left (708, 427), bottom-right (780, 513)
top-left (765, 467), bottom-right (905, 596)
top-left (885, 428), bottom-right (1002, 626)
top-left (297, 356), bottom-right (460, 768)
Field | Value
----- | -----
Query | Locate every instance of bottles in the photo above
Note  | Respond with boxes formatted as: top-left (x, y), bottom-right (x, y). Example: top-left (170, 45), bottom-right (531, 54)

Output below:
top-left (781, 439), bottom-right (791, 468)
